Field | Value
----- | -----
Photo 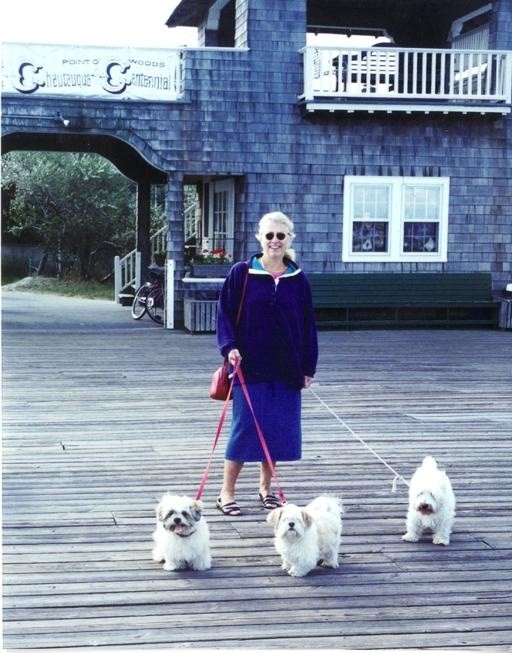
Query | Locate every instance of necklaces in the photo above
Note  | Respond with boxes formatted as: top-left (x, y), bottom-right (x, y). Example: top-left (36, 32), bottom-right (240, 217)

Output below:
top-left (258, 256), bottom-right (284, 272)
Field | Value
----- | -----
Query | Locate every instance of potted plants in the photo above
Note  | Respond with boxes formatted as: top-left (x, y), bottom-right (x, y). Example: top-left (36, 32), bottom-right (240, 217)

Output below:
top-left (154, 252), bottom-right (166, 266)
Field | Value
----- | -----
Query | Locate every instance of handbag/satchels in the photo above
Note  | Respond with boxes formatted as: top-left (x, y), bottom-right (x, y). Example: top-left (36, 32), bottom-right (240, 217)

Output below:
top-left (211, 367), bottom-right (232, 401)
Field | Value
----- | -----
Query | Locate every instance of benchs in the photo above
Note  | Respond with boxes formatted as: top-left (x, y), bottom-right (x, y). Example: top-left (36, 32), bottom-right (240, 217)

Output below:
top-left (303, 274), bottom-right (501, 331)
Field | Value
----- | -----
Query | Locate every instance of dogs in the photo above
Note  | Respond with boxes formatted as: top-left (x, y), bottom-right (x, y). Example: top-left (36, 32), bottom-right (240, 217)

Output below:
top-left (147, 490), bottom-right (212, 574)
top-left (266, 494), bottom-right (343, 580)
top-left (401, 456), bottom-right (457, 546)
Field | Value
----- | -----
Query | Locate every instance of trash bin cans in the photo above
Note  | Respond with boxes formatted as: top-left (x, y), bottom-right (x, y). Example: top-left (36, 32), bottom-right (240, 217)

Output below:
top-left (498, 283), bottom-right (512, 331)
top-left (182, 277), bottom-right (227, 335)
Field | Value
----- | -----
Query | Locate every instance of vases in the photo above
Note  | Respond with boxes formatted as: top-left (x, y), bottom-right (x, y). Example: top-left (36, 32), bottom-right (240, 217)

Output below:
top-left (191, 264), bottom-right (232, 278)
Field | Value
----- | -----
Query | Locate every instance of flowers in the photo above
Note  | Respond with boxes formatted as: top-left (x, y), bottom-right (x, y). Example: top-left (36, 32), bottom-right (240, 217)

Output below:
top-left (193, 248), bottom-right (232, 264)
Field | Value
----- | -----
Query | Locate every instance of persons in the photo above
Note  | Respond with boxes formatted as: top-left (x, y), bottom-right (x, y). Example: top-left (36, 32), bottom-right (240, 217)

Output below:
top-left (214, 210), bottom-right (320, 517)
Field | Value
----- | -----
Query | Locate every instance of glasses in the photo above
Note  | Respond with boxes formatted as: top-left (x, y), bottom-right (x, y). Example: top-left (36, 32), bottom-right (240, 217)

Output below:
top-left (266, 232), bottom-right (286, 241)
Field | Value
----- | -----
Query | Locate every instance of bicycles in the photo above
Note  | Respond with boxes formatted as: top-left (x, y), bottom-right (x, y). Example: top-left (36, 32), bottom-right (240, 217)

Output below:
top-left (131, 268), bottom-right (165, 324)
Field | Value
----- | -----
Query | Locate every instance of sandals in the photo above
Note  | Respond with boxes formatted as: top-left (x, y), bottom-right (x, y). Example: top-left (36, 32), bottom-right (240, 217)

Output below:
top-left (216, 497), bottom-right (241, 515)
top-left (258, 490), bottom-right (280, 509)
